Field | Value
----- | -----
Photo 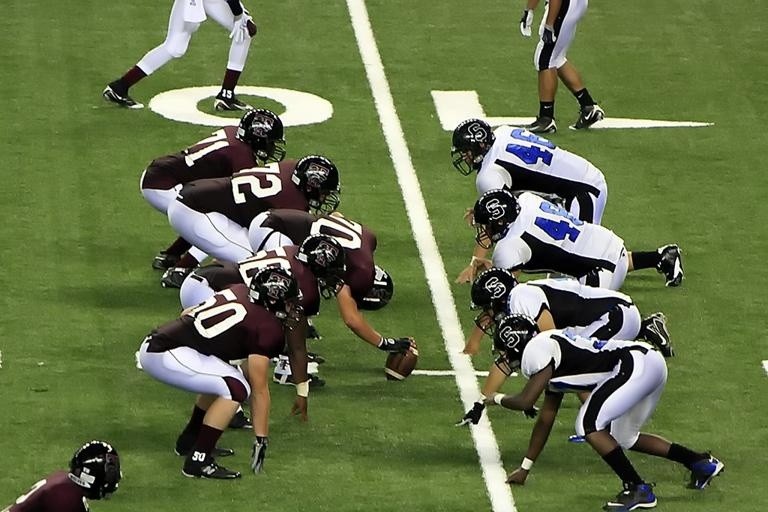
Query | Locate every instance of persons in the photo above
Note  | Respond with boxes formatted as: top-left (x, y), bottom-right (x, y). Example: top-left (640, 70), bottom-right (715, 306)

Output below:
top-left (139, 266), bottom-right (298, 479)
top-left (456, 268), bottom-right (642, 425)
top-left (249, 210), bottom-right (417, 387)
top-left (462, 189), bottom-right (674, 359)
top-left (0, 441), bottom-right (123, 511)
top-left (167, 156), bottom-right (341, 264)
top-left (139, 107), bottom-right (286, 287)
top-left (483, 316), bottom-right (724, 510)
top-left (180, 234), bottom-right (344, 430)
top-left (103, 0), bottom-right (256, 111)
top-left (519, 1), bottom-right (605, 133)
top-left (450, 118), bottom-right (683, 289)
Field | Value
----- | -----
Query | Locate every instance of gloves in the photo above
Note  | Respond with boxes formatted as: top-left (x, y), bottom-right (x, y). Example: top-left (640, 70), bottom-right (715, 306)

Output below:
top-left (519, 8), bottom-right (533, 38)
top-left (250, 435), bottom-right (270, 477)
top-left (229, 19), bottom-right (251, 45)
top-left (378, 332), bottom-right (413, 354)
top-left (522, 405), bottom-right (539, 420)
top-left (542, 24), bottom-right (557, 45)
top-left (456, 394), bottom-right (486, 428)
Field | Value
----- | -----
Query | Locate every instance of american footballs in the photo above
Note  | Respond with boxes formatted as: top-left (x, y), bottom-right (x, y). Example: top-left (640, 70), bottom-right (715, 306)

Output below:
top-left (384, 336), bottom-right (419, 381)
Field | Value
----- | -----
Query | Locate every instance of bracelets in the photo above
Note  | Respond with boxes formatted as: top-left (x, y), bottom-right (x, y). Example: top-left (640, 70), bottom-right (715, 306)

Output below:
top-left (521, 457), bottom-right (533, 471)
top-left (470, 255), bottom-right (478, 266)
top-left (494, 394), bottom-right (505, 407)
top-left (296, 382), bottom-right (309, 398)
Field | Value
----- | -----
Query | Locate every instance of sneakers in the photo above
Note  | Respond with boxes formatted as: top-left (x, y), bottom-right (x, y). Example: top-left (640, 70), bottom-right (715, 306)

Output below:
top-left (684, 451), bottom-right (726, 490)
top-left (102, 85), bottom-right (145, 111)
top-left (154, 253), bottom-right (176, 270)
top-left (569, 103), bottom-right (606, 131)
top-left (235, 413), bottom-right (254, 429)
top-left (273, 365), bottom-right (327, 389)
top-left (521, 115), bottom-right (558, 135)
top-left (174, 436), bottom-right (235, 457)
top-left (213, 89), bottom-right (254, 112)
top-left (643, 312), bottom-right (676, 358)
top-left (181, 457), bottom-right (242, 480)
top-left (602, 480), bottom-right (659, 512)
top-left (567, 434), bottom-right (588, 444)
top-left (656, 241), bottom-right (685, 288)
top-left (273, 345), bottom-right (326, 365)
top-left (160, 262), bottom-right (192, 288)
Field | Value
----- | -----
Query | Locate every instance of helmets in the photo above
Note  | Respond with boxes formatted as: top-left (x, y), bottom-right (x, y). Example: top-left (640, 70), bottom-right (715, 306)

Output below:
top-left (295, 233), bottom-right (350, 300)
top-left (291, 153), bottom-right (342, 216)
top-left (470, 188), bottom-right (521, 250)
top-left (491, 312), bottom-right (541, 377)
top-left (66, 439), bottom-right (123, 501)
top-left (361, 263), bottom-right (394, 311)
top-left (447, 118), bottom-right (495, 176)
top-left (235, 107), bottom-right (288, 164)
top-left (469, 266), bottom-right (518, 339)
top-left (248, 265), bottom-right (299, 323)
